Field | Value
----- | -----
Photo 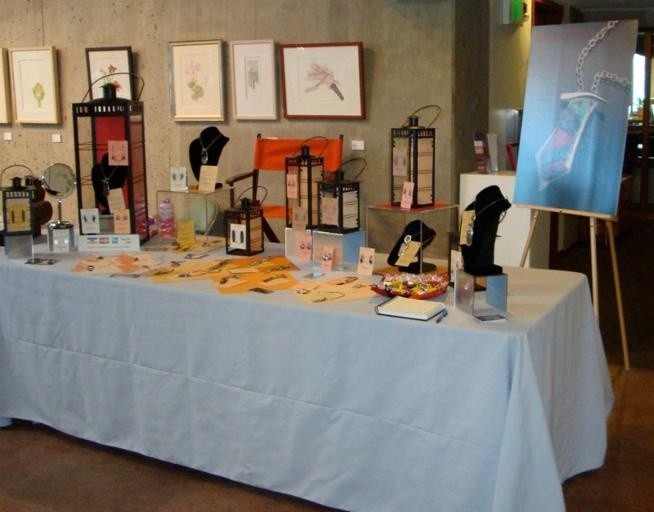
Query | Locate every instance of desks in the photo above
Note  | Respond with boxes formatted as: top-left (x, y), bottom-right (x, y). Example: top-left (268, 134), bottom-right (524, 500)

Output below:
top-left (0, 231), bottom-right (615, 511)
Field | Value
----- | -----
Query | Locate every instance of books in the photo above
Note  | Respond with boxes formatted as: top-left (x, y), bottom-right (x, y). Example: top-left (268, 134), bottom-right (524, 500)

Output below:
top-left (374, 295), bottom-right (445, 320)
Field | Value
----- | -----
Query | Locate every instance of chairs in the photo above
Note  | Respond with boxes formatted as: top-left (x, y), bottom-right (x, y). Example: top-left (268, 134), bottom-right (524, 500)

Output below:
top-left (226, 134), bottom-right (344, 242)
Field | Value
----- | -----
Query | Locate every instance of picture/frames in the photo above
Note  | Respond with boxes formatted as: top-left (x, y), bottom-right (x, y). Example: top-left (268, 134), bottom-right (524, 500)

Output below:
top-left (167, 40), bottom-right (227, 122)
top-left (8, 45), bottom-right (62, 125)
top-left (278, 42), bottom-right (366, 121)
top-left (85, 46), bottom-right (135, 112)
top-left (230, 40), bottom-right (278, 121)
top-left (0, 48), bottom-right (11, 124)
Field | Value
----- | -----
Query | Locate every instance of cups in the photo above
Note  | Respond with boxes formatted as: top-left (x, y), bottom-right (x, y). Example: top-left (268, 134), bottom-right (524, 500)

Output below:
top-left (175, 220), bottom-right (195, 245)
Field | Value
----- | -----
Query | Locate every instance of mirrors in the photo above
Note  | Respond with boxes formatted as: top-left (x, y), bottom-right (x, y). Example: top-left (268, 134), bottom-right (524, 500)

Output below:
top-left (42, 162), bottom-right (76, 229)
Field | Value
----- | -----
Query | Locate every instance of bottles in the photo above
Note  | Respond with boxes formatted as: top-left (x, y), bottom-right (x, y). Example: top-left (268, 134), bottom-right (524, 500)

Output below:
top-left (160, 198), bottom-right (176, 240)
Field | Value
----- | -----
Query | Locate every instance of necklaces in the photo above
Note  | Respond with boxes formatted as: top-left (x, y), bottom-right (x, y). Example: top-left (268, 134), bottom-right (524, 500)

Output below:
top-left (198, 133), bottom-right (222, 165)
top-left (466, 198), bottom-right (501, 245)
top-left (397, 229), bottom-right (425, 256)
top-left (97, 163), bottom-right (120, 197)
